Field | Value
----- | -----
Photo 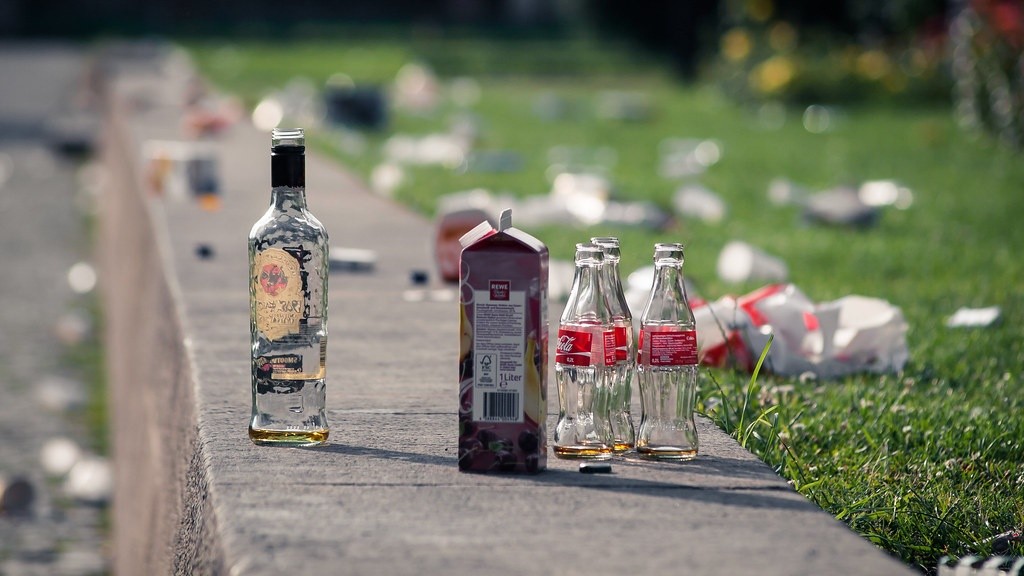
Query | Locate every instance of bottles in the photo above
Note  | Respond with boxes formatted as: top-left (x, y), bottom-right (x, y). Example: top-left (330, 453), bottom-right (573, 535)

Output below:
top-left (551, 242), bottom-right (616, 461)
top-left (588, 236), bottom-right (635, 454)
top-left (247, 127), bottom-right (333, 448)
top-left (633, 242), bottom-right (700, 462)
